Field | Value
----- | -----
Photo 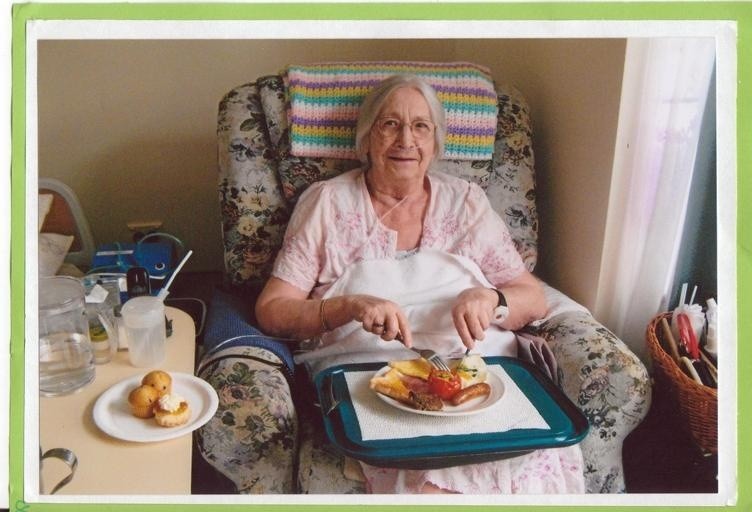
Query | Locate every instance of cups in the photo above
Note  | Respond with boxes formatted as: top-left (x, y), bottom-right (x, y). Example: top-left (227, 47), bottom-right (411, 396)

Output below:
top-left (38, 274), bottom-right (119, 397)
top-left (122, 297), bottom-right (167, 370)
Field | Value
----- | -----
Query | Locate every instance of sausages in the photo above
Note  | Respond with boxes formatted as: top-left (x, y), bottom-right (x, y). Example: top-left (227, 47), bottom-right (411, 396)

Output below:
top-left (450, 383), bottom-right (490, 405)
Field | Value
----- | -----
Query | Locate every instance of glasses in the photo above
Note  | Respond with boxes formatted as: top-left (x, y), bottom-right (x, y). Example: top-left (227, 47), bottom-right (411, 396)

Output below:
top-left (374, 115), bottom-right (436, 140)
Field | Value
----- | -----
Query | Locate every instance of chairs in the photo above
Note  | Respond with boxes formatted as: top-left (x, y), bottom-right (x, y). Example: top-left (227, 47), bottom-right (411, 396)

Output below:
top-left (193, 59), bottom-right (652, 494)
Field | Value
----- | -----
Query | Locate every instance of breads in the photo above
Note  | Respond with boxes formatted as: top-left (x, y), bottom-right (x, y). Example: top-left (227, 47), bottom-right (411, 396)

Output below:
top-left (391, 357), bottom-right (432, 380)
top-left (127, 369), bottom-right (189, 428)
top-left (368, 368), bottom-right (413, 404)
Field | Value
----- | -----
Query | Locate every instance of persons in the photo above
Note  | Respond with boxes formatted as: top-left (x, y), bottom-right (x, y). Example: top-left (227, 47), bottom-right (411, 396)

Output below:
top-left (250, 73), bottom-right (549, 349)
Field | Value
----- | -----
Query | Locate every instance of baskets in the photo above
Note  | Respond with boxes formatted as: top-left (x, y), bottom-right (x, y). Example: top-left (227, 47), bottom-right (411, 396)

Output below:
top-left (645, 310), bottom-right (716, 458)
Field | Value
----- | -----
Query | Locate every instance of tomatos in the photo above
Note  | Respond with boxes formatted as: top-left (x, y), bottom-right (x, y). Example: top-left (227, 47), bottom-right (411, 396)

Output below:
top-left (428, 369), bottom-right (461, 401)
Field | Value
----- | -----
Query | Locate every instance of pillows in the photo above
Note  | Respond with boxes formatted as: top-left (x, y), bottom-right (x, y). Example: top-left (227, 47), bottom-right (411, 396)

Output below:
top-left (38, 193), bottom-right (54, 236)
top-left (37, 230), bottom-right (76, 279)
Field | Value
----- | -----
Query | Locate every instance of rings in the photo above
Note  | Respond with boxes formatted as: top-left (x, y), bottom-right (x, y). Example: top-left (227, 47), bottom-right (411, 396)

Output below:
top-left (372, 321), bottom-right (385, 331)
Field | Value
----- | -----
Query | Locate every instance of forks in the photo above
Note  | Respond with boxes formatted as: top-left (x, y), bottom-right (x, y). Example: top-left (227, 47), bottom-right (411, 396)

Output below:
top-left (395, 336), bottom-right (450, 373)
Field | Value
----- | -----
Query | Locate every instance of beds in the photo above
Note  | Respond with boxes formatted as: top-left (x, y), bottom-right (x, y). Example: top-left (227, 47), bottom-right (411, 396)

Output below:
top-left (37, 178), bottom-right (96, 274)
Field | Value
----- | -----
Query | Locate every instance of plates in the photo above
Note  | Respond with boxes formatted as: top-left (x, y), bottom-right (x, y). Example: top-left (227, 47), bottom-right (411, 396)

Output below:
top-left (91, 371), bottom-right (220, 442)
top-left (370, 364), bottom-right (505, 416)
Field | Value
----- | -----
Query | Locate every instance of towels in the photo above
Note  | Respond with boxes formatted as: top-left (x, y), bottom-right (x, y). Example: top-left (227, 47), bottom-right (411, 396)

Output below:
top-left (195, 288), bottom-right (304, 390)
top-left (293, 250), bottom-right (518, 380)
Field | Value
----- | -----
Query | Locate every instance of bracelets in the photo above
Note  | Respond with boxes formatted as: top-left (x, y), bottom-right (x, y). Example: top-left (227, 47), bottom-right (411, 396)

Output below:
top-left (320, 296), bottom-right (332, 334)
top-left (487, 286), bottom-right (511, 327)
